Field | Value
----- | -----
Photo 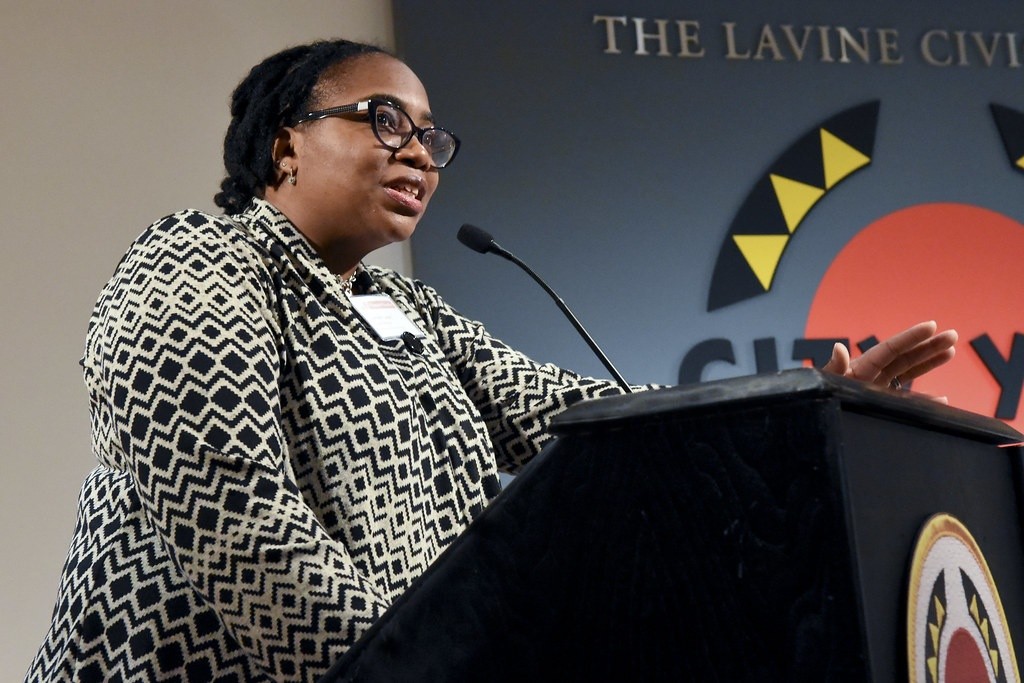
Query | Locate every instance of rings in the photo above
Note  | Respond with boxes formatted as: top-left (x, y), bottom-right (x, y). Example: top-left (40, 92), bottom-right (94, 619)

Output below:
top-left (892, 376), bottom-right (902, 392)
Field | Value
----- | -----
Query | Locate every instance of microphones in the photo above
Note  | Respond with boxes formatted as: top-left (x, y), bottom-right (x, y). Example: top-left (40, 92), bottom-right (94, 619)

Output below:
top-left (456, 221), bottom-right (633, 395)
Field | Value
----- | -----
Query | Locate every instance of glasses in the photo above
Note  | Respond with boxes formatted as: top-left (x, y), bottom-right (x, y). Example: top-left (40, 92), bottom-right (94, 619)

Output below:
top-left (283, 97), bottom-right (462, 169)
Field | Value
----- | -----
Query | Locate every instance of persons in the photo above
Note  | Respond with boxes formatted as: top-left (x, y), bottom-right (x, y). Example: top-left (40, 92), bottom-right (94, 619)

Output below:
top-left (24, 40), bottom-right (959, 683)
